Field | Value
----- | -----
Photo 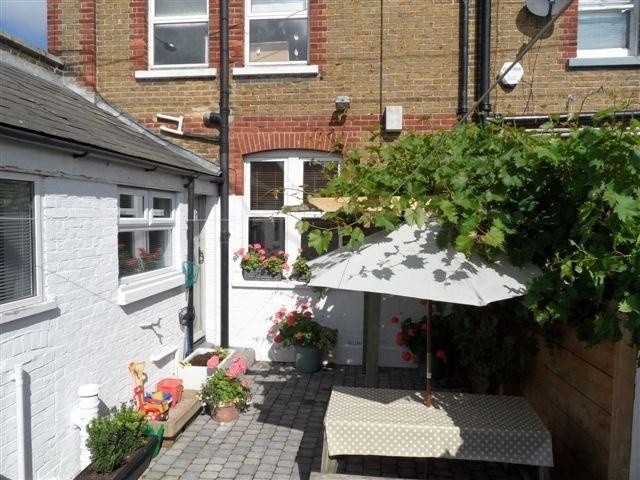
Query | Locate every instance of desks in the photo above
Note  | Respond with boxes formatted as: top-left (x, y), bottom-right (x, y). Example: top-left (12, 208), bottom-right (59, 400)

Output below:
top-left (321, 387), bottom-right (553, 480)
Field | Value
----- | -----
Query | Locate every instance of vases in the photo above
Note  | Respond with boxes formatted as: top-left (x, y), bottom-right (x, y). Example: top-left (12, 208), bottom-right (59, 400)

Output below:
top-left (243, 269), bottom-right (309, 282)
top-left (208, 402), bottom-right (239, 422)
top-left (419, 357), bottom-right (445, 379)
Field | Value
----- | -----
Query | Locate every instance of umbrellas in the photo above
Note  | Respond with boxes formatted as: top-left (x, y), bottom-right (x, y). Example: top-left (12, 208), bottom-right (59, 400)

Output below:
top-left (297, 207), bottom-right (544, 480)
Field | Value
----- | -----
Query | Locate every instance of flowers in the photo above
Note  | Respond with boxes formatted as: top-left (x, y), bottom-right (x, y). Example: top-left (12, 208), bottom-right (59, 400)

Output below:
top-left (118, 242), bottom-right (166, 274)
top-left (266, 298), bottom-right (338, 358)
top-left (194, 352), bottom-right (254, 414)
top-left (388, 299), bottom-right (466, 363)
top-left (234, 242), bottom-right (311, 276)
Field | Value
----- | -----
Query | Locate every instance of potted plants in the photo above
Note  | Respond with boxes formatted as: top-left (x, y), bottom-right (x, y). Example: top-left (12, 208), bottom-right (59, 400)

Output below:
top-left (71, 401), bottom-right (164, 480)
